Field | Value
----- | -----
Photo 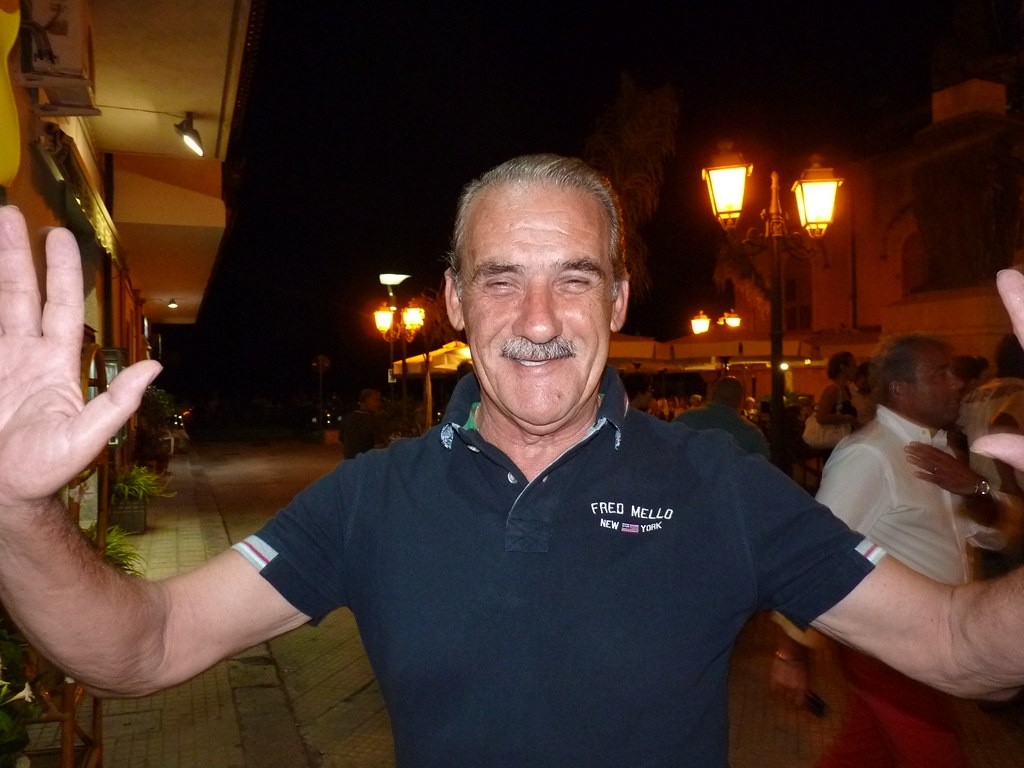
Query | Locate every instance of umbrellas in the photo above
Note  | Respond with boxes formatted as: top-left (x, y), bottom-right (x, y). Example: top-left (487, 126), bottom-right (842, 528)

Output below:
top-left (386, 322), bottom-right (830, 403)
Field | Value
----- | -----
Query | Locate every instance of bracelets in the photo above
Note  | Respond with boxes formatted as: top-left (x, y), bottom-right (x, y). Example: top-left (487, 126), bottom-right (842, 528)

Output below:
top-left (775, 650), bottom-right (808, 666)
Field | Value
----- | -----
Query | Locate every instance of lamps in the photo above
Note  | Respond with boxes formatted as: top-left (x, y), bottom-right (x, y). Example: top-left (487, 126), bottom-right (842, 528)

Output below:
top-left (167, 298), bottom-right (178, 308)
top-left (174, 110), bottom-right (205, 157)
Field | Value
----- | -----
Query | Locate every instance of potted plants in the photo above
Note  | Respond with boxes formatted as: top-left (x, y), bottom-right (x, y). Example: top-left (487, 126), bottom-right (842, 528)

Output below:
top-left (108, 463), bottom-right (178, 534)
top-left (136, 428), bottom-right (168, 472)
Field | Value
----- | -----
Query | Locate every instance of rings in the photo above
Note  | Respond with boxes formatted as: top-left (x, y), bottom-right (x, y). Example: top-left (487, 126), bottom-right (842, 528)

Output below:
top-left (934, 466), bottom-right (942, 474)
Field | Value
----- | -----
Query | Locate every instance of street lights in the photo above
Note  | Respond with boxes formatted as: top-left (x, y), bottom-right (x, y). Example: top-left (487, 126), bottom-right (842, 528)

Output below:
top-left (696, 137), bottom-right (846, 478)
top-left (373, 298), bottom-right (426, 437)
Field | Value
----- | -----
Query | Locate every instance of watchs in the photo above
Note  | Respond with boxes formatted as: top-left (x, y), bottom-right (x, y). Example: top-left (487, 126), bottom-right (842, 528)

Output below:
top-left (962, 478), bottom-right (993, 513)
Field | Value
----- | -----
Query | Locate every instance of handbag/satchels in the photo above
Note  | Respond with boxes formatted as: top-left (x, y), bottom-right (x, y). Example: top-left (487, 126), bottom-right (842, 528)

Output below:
top-left (801, 383), bottom-right (852, 449)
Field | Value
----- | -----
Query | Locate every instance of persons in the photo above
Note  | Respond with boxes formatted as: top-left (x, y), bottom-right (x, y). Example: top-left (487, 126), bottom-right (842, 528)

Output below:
top-left (816, 333), bottom-right (1024, 745)
top-left (619, 368), bottom-right (820, 463)
top-left (0, 154), bottom-right (1023, 768)
top-left (765, 333), bottom-right (1024, 768)
top-left (338, 389), bottom-right (387, 459)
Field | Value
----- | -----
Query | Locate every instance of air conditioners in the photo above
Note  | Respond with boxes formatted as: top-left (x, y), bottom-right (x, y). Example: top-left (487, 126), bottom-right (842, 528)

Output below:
top-left (21, 0), bottom-right (102, 116)
